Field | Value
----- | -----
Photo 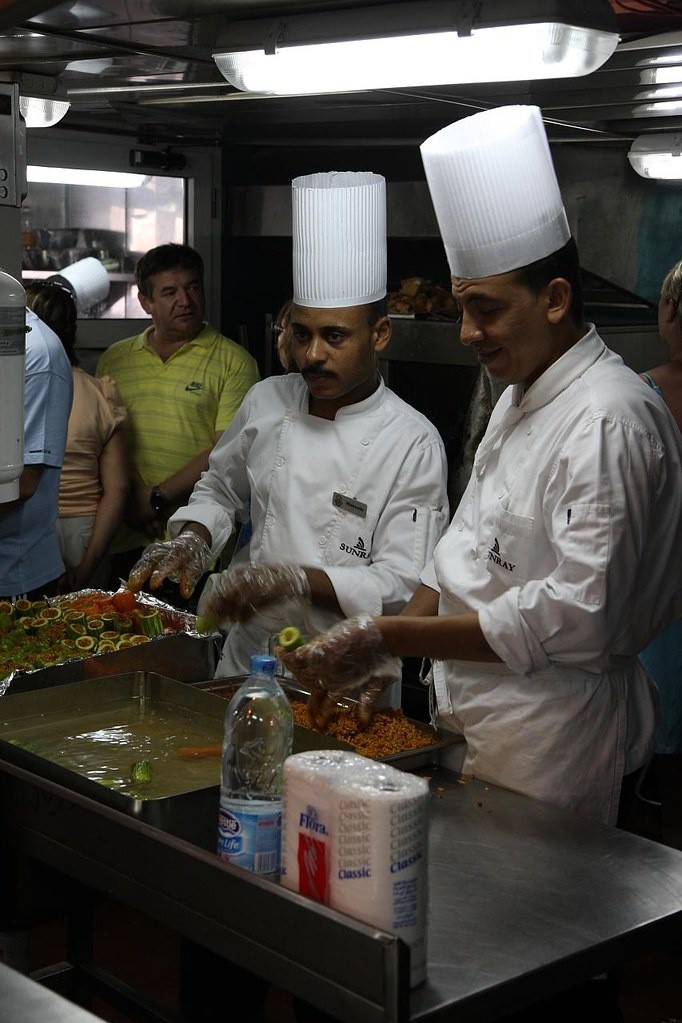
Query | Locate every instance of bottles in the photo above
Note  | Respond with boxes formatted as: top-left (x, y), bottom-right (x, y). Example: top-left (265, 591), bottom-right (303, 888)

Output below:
top-left (218, 653), bottom-right (298, 883)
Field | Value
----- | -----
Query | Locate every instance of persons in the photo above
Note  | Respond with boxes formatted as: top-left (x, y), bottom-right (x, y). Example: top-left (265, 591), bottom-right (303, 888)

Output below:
top-left (124, 289), bottom-right (450, 730)
top-left (0, 304), bottom-right (69, 602)
top-left (272, 239), bottom-right (682, 848)
top-left (23, 279), bottom-right (132, 594)
top-left (90, 244), bottom-right (260, 658)
top-left (631, 258), bottom-right (682, 755)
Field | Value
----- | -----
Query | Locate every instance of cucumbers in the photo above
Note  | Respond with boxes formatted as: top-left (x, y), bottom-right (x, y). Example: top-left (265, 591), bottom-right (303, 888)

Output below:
top-left (131, 761), bottom-right (153, 786)
top-left (279, 626), bottom-right (306, 651)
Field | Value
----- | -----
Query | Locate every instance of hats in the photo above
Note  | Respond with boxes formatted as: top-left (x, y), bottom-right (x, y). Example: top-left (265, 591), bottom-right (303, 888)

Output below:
top-left (290, 169), bottom-right (390, 310)
top-left (419, 103), bottom-right (572, 282)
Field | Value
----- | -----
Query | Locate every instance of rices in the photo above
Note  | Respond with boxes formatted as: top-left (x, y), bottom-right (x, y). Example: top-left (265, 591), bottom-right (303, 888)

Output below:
top-left (288, 697), bottom-right (436, 761)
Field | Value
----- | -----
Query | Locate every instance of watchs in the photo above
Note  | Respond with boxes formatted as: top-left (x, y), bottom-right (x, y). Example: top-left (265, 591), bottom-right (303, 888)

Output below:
top-left (149, 485), bottom-right (174, 515)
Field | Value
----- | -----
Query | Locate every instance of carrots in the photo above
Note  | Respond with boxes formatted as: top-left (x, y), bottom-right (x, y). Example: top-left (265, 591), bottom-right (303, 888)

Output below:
top-left (175, 745), bottom-right (220, 761)
top-left (68, 589), bottom-right (136, 618)
